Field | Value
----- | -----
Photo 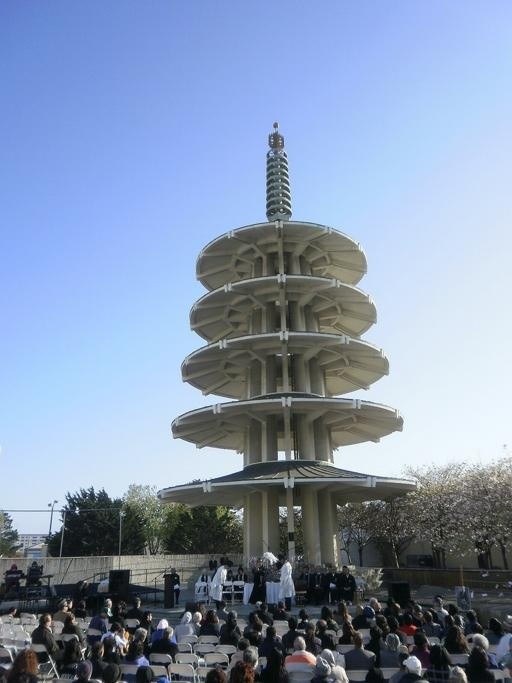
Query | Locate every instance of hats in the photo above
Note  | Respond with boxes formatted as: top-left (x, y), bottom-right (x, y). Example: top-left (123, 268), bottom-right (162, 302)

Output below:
top-left (403, 655), bottom-right (422, 670)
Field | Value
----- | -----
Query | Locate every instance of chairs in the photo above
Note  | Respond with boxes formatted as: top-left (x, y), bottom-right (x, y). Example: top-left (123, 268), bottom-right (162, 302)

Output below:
top-left (0, 613), bottom-right (512, 682)
top-left (456, 586), bottom-right (473, 620)
top-left (193, 571), bottom-right (367, 609)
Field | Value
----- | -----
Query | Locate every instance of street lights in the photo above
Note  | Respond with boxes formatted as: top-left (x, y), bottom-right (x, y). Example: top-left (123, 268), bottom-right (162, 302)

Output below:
top-left (46, 498), bottom-right (60, 540)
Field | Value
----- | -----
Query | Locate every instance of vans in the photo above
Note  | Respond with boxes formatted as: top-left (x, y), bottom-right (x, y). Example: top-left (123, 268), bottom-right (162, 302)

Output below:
top-left (406, 553), bottom-right (434, 568)
